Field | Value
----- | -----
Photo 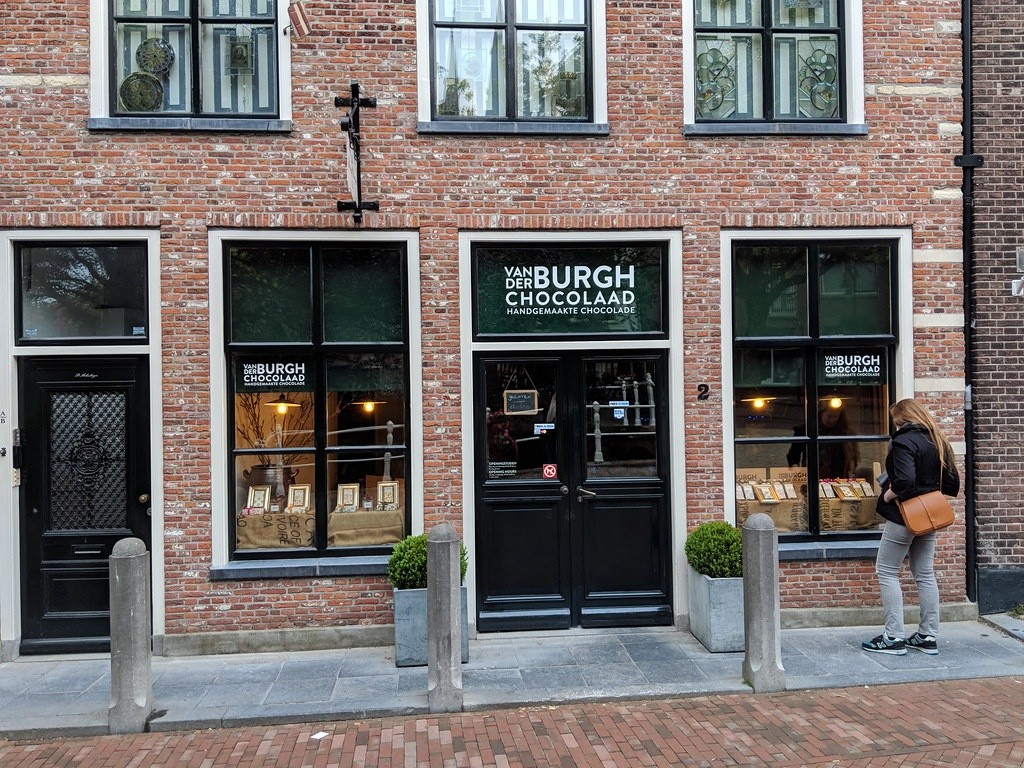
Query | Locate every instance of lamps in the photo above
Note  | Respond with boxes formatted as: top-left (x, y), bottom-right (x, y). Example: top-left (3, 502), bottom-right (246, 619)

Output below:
top-left (741, 393), bottom-right (777, 409)
top-left (350, 397), bottom-right (387, 413)
top-left (819, 394), bottom-right (852, 409)
top-left (265, 394), bottom-right (300, 415)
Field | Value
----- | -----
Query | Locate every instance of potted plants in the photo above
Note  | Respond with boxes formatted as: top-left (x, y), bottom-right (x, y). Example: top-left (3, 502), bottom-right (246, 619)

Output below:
top-left (388, 535), bottom-right (470, 665)
top-left (685, 520), bottom-right (749, 655)
top-left (238, 394), bottom-right (354, 499)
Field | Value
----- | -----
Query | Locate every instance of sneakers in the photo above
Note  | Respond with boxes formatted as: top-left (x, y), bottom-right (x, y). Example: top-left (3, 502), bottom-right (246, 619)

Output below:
top-left (904, 632), bottom-right (939, 654)
top-left (862, 632), bottom-right (907, 655)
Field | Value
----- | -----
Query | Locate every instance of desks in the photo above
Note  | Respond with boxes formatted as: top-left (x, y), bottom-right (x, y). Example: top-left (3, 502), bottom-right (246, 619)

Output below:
top-left (819, 499), bottom-right (878, 532)
top-left (329, 507), bottom-right (404, 546)
top-left (737, 497), bottom-right (805, 531)
top-left (237, 493), bottom-right (316, 551)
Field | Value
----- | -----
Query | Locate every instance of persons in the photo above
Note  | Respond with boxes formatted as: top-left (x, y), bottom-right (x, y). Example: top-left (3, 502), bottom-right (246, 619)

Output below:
top-left (787, 392), bottom-right (857, 480)
top-left (857, 397), bottom-right (960, 654)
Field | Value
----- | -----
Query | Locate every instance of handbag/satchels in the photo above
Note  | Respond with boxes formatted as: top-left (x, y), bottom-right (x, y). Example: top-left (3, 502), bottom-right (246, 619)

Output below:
top-left (899, 490), bottom-right (956, 537)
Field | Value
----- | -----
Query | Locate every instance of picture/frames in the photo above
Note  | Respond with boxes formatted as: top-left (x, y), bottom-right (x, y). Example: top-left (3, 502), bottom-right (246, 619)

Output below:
top-left (247, 485), bottom-right (271, 511)
top-left (833, 483), bottom-right (862, 502)
top-left (754, 484), bottom-right (781, 504)
top-left (288, 484), bottom-right (311, 510)
top-left (337, 483), bottom-right (359, 509)
top-left (377, 481), bottom-right (400, 508)
